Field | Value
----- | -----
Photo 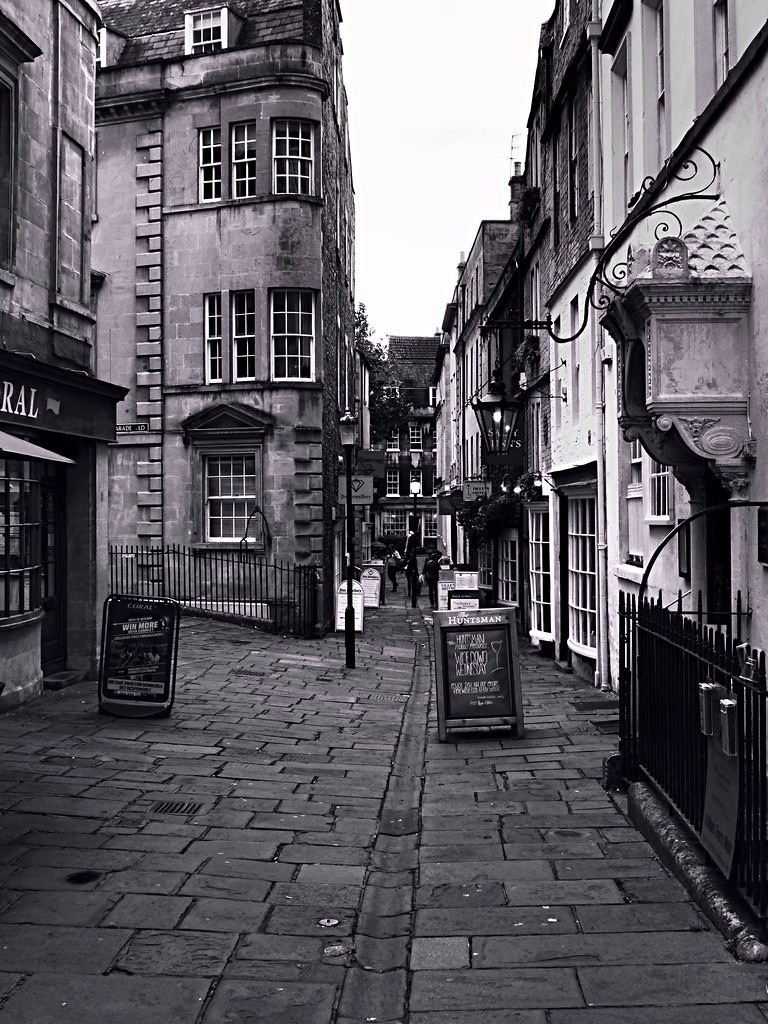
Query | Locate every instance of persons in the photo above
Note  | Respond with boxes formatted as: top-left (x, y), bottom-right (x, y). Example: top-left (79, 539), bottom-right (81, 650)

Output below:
top-left (387, 544), bottom-right (441, 607)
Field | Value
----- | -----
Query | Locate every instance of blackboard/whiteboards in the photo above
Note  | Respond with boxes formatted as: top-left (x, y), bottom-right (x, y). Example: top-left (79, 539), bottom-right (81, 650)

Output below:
top-left (436, 611), bottom-right (523, 726)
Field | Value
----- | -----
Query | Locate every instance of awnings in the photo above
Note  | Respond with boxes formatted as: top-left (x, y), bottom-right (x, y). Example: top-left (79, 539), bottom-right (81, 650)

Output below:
top-left (0, 431), bottom-right (76, 464)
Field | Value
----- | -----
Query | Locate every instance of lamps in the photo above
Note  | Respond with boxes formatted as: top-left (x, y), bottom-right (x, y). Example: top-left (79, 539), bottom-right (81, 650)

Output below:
top-left (470, 145), bottom-right (720, 457)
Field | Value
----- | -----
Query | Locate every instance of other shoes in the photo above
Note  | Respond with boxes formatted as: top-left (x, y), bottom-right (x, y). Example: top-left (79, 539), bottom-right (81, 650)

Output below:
top-left (430, 605), bottom-right (434, 608)
